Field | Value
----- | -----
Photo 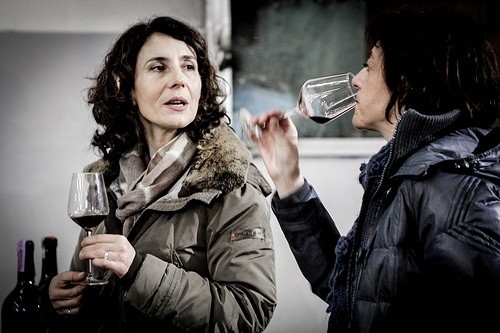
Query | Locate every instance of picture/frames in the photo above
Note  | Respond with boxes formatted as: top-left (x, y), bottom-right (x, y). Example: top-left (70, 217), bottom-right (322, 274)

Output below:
top-left (204, 0), bottom-right (500, 158)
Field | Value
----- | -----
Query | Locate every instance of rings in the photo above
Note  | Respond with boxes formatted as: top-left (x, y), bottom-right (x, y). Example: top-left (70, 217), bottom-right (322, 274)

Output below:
top-left (104, 249), bottom-right (109, 260)
top-left (68, 308), bottom-right (71, 314)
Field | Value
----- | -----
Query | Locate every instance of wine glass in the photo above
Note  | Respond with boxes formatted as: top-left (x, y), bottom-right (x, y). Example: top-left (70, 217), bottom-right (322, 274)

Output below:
top-left (238, 72), bottom-right (359, 145)
top-left (67, 172), bottom-right (110, 285)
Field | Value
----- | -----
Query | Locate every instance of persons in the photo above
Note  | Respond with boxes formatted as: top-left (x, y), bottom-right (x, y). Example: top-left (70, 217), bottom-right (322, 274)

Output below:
top-left (247, 0), bottom-right (500, 333)
top-left (48, 15), bottom-right (277, 333)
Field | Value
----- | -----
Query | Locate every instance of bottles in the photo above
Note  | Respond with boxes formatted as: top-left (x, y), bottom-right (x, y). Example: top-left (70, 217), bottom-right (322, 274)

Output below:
top-left (37, 237), bottom-right (60, 333)
top-left (1, 240), bottom-right (38, 333)
